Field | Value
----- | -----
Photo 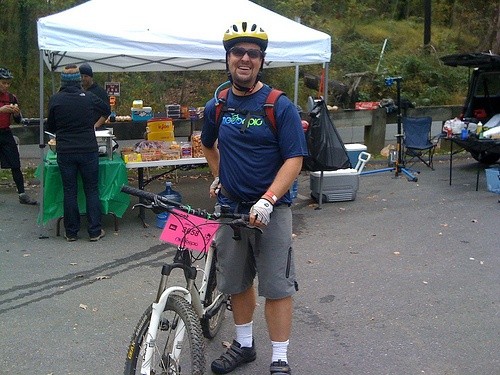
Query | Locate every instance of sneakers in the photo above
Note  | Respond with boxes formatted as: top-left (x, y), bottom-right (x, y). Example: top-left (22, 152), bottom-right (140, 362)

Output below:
top-left (269, 359), bottom-right (291, 375)
top-left (211, 338), bottom-right (257, 373)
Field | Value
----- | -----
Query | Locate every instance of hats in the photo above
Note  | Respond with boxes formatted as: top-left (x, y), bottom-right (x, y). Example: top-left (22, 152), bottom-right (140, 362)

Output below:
top-left (61, 64), bottom-right (81, 81)
top-left (79, 63), bottom-right (93, 77)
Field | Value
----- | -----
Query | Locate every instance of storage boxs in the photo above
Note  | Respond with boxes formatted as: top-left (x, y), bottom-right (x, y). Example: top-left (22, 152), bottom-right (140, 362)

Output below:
top-left (484, 168), bottom-right (500, 193)
top-left (345, 144), bottom-right (367, 169)
top-left (313, 170), bottom-right (359, 204)
top-left (131, 107), bottom-right (153, 121)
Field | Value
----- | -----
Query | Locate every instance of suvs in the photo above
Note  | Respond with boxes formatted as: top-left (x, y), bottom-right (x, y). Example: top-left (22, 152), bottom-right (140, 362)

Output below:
top-left (439, 51), bottom-right (500, 165)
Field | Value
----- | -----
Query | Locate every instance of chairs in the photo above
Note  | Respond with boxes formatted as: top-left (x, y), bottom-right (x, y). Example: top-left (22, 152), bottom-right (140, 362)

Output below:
top-left (395, 116), bottom-right (436, 169)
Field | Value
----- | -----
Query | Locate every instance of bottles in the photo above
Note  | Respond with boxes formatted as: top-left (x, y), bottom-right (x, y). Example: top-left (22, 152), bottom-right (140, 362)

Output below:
top-left (477, 121), bottom-right (482, 135)
top-left (288, 177), bottom-right (299, 198)
top-left (144, 126), bottom-right (148, 140)
top-left (155, 182), bottom-right (181, 229)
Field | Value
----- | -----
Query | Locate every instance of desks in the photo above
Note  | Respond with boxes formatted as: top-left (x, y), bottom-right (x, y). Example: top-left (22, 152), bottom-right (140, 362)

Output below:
top-left (444, 136), bottom-right (500, 192)
top-left (33, 144), bottom-right (130, 238)
top-left (126, 157), bottom-right (209, 229)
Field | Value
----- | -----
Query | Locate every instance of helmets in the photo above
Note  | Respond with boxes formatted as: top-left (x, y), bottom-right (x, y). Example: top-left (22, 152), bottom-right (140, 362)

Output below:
top-left (223, 21), bottom-right (268, 52)
top-left (0, 67), bottom-right (13, 78)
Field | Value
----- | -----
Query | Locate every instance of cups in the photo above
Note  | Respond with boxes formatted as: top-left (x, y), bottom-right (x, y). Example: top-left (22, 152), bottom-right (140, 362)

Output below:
top-left (178, 136), bottom-right (188, 144)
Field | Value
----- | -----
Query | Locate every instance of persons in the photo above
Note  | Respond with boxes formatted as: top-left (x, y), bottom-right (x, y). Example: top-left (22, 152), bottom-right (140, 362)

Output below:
top-left (47, 63), bottom-right (109, 242)
top-left (0, 68), bottom-right (39, 205)
top-left (79, 62), bottom-right (111, 131)
top-left (199, 20), bottom-right (308, 375)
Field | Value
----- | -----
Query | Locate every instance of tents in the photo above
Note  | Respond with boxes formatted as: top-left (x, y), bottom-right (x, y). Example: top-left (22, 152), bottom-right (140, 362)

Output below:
top-left (36, 0), bottom-right (331, 237)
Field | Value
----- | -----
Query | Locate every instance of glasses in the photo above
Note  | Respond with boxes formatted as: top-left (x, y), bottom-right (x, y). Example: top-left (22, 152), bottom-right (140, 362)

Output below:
top-left (227, 47), bottom-right (263, 58)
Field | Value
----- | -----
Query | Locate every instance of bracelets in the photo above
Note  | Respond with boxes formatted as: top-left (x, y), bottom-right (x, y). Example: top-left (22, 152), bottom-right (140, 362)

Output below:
top-left (265, 191), bottom-right (278, 203)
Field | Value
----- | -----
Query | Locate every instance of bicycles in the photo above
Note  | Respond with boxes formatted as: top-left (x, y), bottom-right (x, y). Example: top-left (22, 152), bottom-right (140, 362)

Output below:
top-left (120, 183), bottom-right (264, 375)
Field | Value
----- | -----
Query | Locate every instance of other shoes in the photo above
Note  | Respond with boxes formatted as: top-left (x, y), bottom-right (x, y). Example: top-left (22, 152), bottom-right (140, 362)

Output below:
top-left (64, 231), bottom-right (77, 241)
top-left (90, 229), bottom-right (105, 240)
top-left (18, 194), bottom-right (37, 204)
